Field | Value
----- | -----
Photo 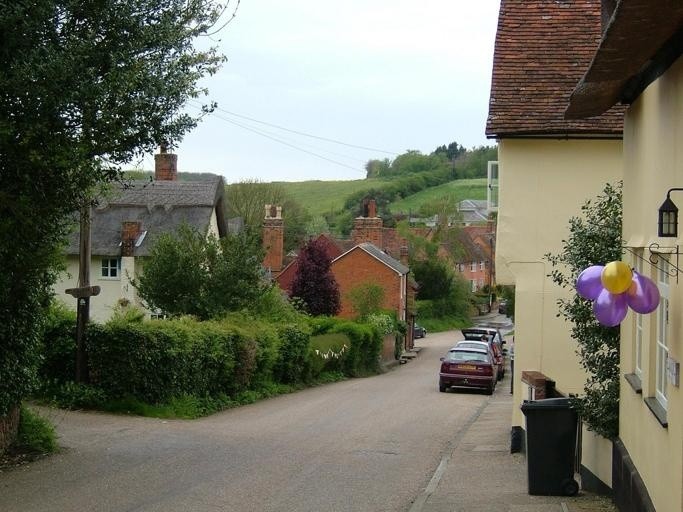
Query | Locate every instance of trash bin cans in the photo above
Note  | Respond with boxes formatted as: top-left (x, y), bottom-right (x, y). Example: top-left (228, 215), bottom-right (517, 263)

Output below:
top-left (520, 398), bottom-right (583, 497)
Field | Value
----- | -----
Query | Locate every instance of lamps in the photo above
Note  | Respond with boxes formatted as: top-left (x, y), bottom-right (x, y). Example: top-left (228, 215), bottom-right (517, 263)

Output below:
top-left (657, 188), bottom-right (682, 238)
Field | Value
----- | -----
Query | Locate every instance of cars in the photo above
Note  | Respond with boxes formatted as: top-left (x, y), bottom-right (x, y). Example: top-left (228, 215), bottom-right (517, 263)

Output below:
top-left (439, 326), bottom-right (508, 395)
top-left (498, 298), bottom-right (508, 314)
top-left (414, 323), bottom-right (427, 338)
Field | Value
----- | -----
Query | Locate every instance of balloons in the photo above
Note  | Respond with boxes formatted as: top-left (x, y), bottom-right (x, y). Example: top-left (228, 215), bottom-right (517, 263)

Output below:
top-left (577, 265), bottom-right (605, 300)
top-left (625, 269), bottom-right (661, 314)
top-left (593, 287), bottom-right (628, 328)
top-left (602, 261), bottom-right (633, 294)
